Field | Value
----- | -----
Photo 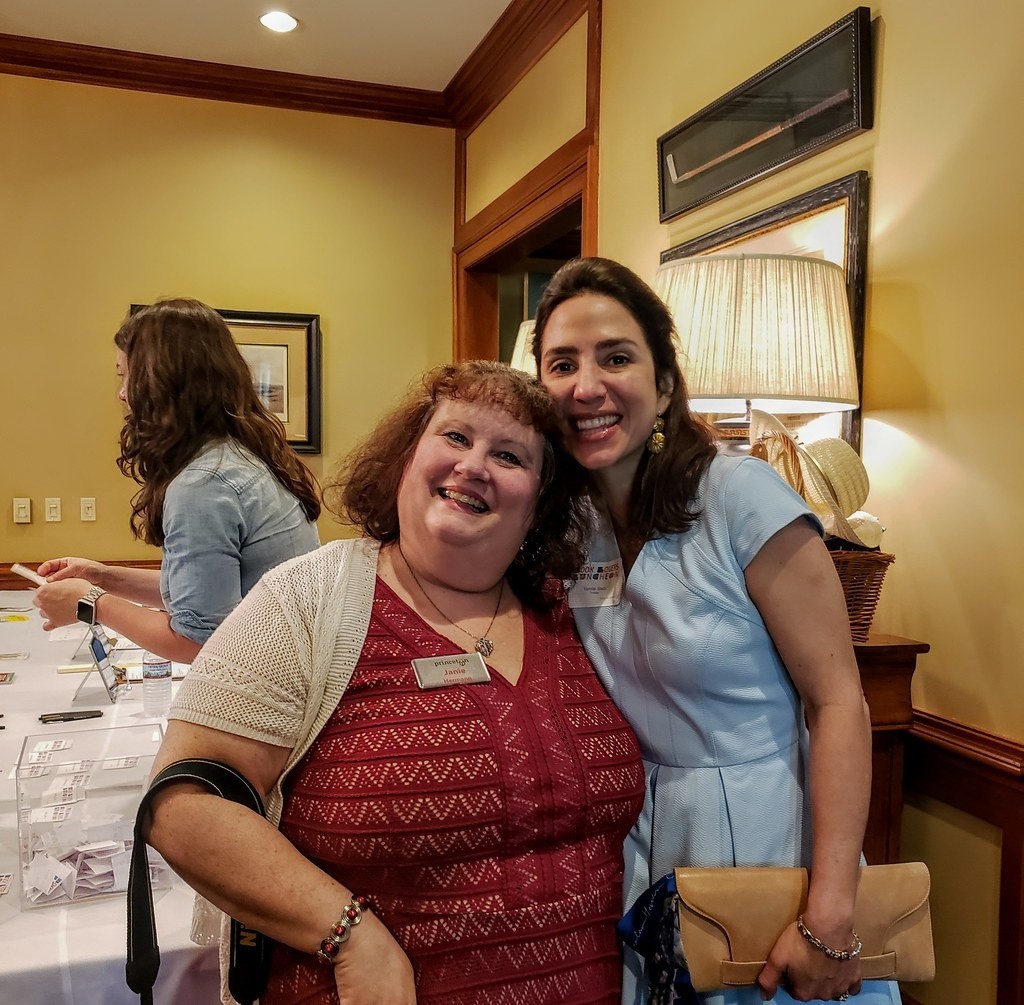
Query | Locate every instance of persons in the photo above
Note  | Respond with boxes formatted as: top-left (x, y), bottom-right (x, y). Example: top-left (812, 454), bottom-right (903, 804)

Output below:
top-left (32, 298), bottom-right (321, 662)
top-left (142, 361), bottom-right (645, 1005)
top-left (530, 258), bottom-right (904, 1005)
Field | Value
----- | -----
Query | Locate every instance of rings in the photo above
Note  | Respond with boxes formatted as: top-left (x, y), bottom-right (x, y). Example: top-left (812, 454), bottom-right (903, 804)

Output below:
top-left (839, 991), bottom-right (848, 1001)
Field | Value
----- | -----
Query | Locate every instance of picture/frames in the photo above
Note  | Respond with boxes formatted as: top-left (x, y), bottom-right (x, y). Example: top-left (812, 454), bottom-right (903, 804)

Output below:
top-left (658, 166), bottom-right (867, 452)
top-left (130, 304), bottom-right (322, 457)
top-left (658, 7), bottom-right (869, 223)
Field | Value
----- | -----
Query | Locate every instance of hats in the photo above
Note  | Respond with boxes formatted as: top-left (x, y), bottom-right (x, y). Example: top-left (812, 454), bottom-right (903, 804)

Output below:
top-left (749, 409), bottom-right (883, 549)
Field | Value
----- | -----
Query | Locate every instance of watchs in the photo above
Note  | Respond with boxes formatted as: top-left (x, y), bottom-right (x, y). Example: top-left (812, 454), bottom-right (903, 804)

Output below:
top-left (76, 587), bottom-right (107, 626)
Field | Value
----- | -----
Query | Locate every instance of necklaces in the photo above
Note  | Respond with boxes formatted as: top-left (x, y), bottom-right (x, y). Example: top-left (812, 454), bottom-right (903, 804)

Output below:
top-left (398, 540), bottom-right (505, 657)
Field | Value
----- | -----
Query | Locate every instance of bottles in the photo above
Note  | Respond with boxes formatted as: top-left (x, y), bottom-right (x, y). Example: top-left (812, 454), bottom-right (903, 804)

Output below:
top-left (141, 650), bottom-right (173, 719)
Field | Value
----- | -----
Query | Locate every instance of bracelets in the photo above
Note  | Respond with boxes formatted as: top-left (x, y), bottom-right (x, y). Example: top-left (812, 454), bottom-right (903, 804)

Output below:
top-left (316, 895), bottom-right (369, 966)
top-left (797, 915), bottom-right (862, 960)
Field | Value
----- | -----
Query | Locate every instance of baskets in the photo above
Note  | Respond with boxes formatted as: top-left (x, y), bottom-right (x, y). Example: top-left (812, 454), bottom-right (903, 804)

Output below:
top-left (750, 430), bottom-right (894, 644)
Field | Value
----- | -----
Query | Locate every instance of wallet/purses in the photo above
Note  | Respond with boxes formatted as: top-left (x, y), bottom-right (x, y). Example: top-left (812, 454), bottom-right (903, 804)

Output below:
top-left (673, 862), bottom-right (934, 992)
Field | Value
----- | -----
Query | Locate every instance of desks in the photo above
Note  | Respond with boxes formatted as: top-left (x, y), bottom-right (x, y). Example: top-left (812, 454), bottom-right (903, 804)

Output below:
top-left (843, 630), bottom-right (929, 863)
top-left (1, 590), bottom-right (227, 1005)
